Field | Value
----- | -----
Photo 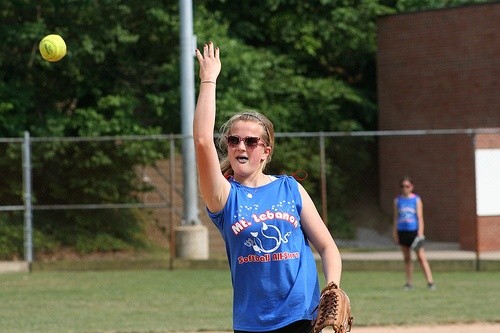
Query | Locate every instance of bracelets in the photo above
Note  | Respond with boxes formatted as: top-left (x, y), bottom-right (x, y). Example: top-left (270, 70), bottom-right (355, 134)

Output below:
top-left (201, 80), bottom-right (216, 83)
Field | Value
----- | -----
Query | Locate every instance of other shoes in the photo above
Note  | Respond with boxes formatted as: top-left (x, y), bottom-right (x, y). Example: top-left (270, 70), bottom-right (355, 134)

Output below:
top-left (428, 283), bottom-right (435, 291)
top-left (403, 285), bottom-right (414, 291)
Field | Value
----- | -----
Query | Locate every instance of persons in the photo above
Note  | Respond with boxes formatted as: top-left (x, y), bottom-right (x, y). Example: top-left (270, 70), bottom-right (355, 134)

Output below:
top-left (393, 178), bottom-right (436, 292)
top-left (193, 42), bottom-right (351, 333)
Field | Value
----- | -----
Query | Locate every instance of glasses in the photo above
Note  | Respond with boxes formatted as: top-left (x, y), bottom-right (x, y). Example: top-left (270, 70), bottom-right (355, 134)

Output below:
top-left (226, 136), bottom-right (268, 150)
top-left (401, 184), bottom-right (411, 189)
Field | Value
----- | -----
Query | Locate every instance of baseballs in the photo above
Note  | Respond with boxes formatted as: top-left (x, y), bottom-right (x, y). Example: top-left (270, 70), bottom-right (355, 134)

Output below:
top-left (37, 33), bottom-right (68, 61)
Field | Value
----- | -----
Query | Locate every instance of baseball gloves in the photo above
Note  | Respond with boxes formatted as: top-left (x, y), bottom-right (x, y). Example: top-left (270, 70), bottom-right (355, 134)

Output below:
top-left (317, 281), bottom-right (353, 333)
top-left (410, 236), bottom-right (426, 250)
